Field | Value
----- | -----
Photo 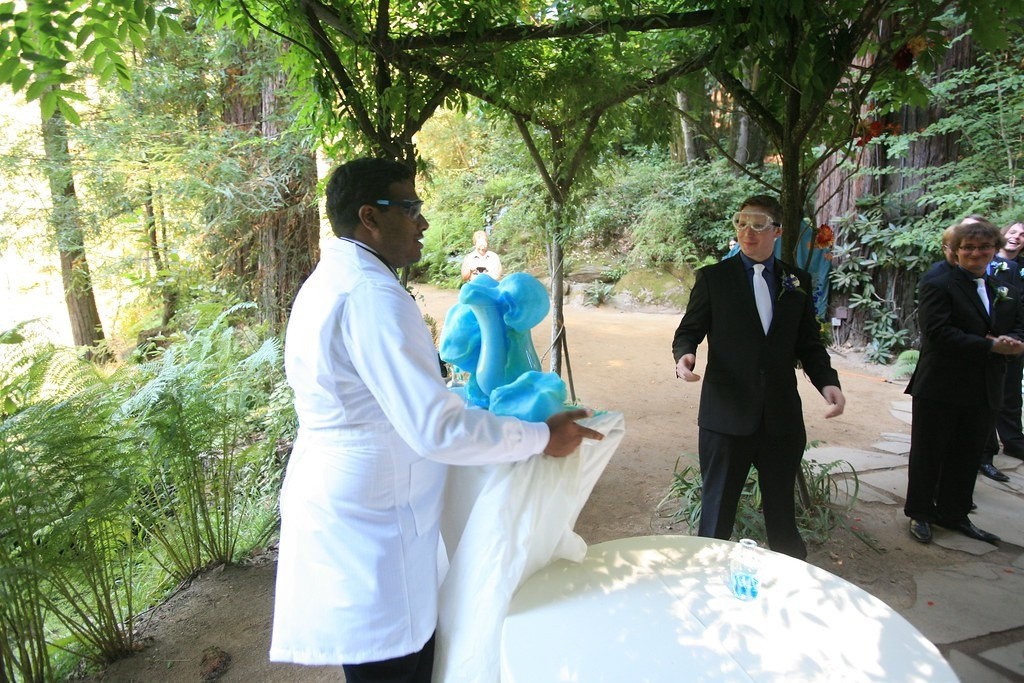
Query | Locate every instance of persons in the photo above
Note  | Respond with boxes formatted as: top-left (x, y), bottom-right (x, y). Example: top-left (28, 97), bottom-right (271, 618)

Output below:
top-left (267, 159), bottom-right (604, 683)
top-left (672, 194), bottom-right (846, 560)
top-left (728, 237), bottom-right (738, 250)
top-left (461, 230), bottom-right (502, 281)
top-left (903, 216), bottom-right (1024, 544)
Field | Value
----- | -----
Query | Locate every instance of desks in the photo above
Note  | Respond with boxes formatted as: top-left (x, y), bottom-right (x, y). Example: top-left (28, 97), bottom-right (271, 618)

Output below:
top-left (499, 534), bottom-right (961, 683)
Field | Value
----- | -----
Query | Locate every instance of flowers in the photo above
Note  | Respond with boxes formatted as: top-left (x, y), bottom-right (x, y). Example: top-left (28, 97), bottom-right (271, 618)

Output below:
top-left (778, 274), bottom-right (808, 301)
top-left (993, 261), bottom-right (1010, 276)
top-left (990, 283), bottom-right (1013, 309)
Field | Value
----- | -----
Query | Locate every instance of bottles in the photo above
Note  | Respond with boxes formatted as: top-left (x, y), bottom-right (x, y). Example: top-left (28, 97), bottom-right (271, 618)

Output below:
top-left (729, 538), bottom-right (761, 601)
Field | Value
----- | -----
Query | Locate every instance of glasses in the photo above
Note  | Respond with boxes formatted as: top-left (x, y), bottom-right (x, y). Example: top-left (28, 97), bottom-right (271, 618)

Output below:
top-left (373, 199), bottom-right (424, 222)
top-left (732, 212), bottom-right (782, 233)
top-left (958, 245), bottom-right (997, 251)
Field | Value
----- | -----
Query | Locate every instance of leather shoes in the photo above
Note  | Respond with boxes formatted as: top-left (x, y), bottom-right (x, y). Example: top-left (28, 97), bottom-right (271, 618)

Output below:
top-left (910, 518), bottom-right (931, 542)
top-left (951, 521), bottom-right (998, 540)
top-left (979, 463), bottom-right (1010, 480)
top-left (970, 503), bottom-right (977, 509)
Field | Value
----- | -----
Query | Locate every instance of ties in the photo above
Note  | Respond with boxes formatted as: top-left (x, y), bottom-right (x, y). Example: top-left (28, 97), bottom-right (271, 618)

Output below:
top-left (973, 278), bottom-right (990, 316)
top-left (753, 264), bottom-right (774, 335)
